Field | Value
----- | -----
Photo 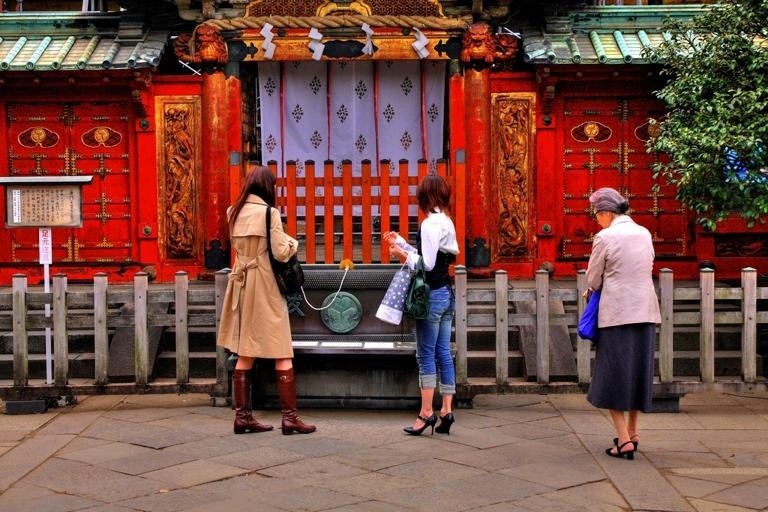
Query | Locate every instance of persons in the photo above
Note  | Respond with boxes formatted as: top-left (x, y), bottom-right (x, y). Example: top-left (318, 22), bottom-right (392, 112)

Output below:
top-left (580, 187), bottom-right (665, 461)
top-left (383, 173), bottom-right (461, 436)
top-left (216, 162), bottom-right (317, 436)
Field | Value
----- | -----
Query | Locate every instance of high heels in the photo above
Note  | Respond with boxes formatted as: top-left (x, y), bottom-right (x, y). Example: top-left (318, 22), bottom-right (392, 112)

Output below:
top-left (605, 441), bottom-right (635, 460)
top-left (435, 412), bottom-right (456, 435)
top-left (401, 411), bottom-right (438, 436)
top-left (613, 433), bottom-right (641, 451)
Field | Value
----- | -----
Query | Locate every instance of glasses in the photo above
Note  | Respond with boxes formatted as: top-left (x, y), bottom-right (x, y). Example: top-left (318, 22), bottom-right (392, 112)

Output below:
top-left (590, 210), bottom-right (600, 218)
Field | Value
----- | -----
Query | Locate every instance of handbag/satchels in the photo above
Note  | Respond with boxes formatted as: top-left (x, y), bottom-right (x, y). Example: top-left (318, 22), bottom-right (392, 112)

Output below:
top-left (265, 205), bottom-right (305, 296)
top-left (402, 255), bottom-right (431, 323)
top-left (373, 252), bottom-right (416, 326)
top-left (576, 289), bottom-right (601, 341)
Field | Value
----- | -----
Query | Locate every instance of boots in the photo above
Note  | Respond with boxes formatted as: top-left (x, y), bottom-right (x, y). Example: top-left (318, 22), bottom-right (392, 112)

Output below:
top-left (275, 366), bottom-right (316, 436)
top-left (232, 365), bottom-right (273, 434)
